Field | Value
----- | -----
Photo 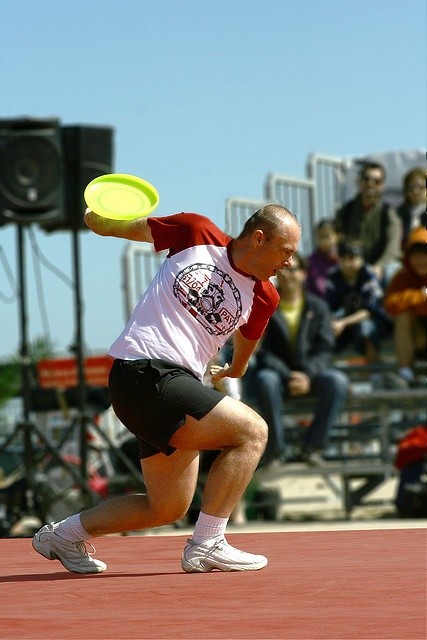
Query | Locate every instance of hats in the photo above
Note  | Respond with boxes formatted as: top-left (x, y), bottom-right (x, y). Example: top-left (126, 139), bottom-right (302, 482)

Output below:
top-left (337, 238), bottom-right (364, 260)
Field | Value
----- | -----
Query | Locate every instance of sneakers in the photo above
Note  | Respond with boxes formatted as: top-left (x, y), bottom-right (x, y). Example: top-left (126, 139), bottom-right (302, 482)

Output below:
top-left (299, 444), bottom-right (326, 468)
top-left (263, 449), bottom-right (285, 471)
top-left (180, 536), bottom-right (269, 573)
top-left (32, 521), bottom-right (107, 575)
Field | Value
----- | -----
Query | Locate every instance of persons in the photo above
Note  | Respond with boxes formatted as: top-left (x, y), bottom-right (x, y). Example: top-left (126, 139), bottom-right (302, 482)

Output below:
top-left (30, 203), bottom-right (303, 575)
top-left (304, 218), bottom-right (344, 298)
top-left (383, 225), bottom-right (426, 386)
top-left (318, 237), bottom-right (396, 388)
top-left (245, 248), bottom-right (351, 470)
top-left (331, 162), bottom-right (407, 292)
top-left (394, 167), bottom-right (426, 252)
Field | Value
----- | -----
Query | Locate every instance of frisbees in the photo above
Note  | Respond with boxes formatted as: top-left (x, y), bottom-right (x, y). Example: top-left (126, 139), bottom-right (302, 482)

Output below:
top-left (83, 174), bottom-right (160, 221)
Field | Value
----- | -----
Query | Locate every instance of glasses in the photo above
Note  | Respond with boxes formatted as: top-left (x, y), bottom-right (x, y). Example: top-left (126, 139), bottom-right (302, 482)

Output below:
top-left (360, 172), bottom-right (385, 185)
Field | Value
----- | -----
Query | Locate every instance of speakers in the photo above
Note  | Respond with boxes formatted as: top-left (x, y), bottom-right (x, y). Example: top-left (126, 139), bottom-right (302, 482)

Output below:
top-left (1, 119), bottom-right (66, 226)
top-left (45, 125), bottom-right (114, 232)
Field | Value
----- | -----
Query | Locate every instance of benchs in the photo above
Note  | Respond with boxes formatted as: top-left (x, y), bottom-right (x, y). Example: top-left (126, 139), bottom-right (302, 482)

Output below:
top-left (233, 391), bottom-right (426, 465)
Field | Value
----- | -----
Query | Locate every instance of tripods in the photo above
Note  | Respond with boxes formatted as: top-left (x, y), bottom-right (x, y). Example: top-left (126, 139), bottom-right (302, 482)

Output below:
top-left (34, 230), bottom-right (147, 500)
top-left (0, 227), bottom-right (95, 521)
